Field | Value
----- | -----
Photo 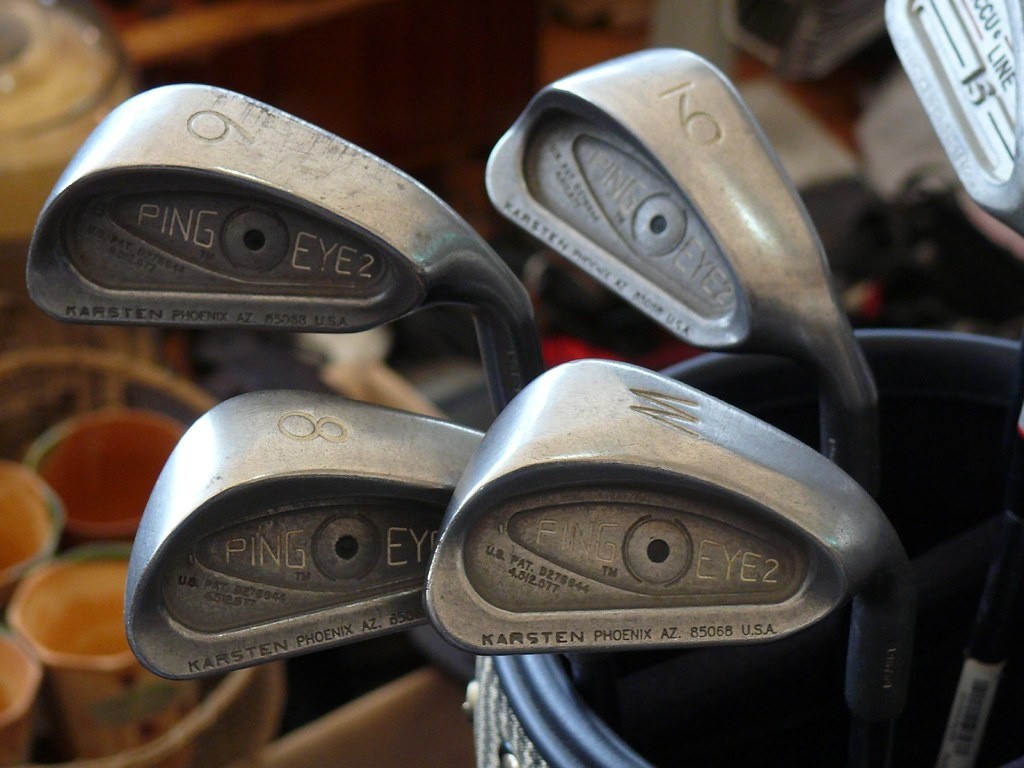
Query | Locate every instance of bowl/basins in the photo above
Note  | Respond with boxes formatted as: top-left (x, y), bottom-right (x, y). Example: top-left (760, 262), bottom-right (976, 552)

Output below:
top-left (0, 344), bottom-right (276, 768)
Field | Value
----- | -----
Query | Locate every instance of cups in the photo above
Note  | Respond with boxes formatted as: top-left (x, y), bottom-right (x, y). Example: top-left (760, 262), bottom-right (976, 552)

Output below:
top-left (25, 410), bottom-right (186, 538)
top-left (0, 627), bottom-right (43, 768)
top-left (0, 460), bottom-right (62, 607)
top-left (0, 0), bottom-right (144, 350)
top-left (9, 545), bottom-right (198, 758)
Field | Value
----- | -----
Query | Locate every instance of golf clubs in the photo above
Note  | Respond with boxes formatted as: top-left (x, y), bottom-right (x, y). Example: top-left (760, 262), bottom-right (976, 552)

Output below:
top-left (125, 389), bottom-right (488, 674)
top-left (884, 0), bottom-right (1024, 234)
top-left (24, 83), bottom-right (542, 415)
top-left (427, 357), bottom-right (897, 714)
top-left (486, 50), bottom-right (886, 496)
